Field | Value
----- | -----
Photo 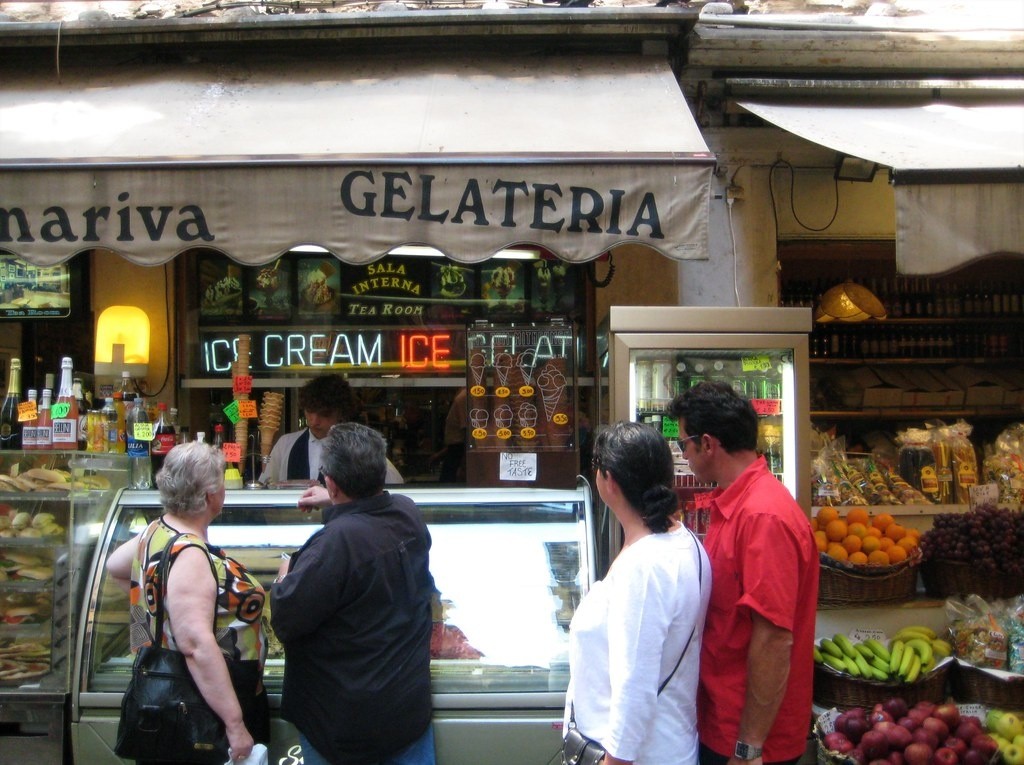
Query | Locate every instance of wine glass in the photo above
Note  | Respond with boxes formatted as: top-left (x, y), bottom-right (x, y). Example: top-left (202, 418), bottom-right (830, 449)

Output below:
top-left (259, 287), bottom-right (278, 307)
top-left (490, 284), bottom-right (515, 305)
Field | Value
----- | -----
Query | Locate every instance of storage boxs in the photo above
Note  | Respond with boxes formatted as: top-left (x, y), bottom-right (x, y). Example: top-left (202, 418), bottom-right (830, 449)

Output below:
top-left (843, 366), bottom-right (903, 408)
top-left (992, 369), bottom-right (1024, 405)
top-left (945, 367), bottom-right (1004, 408)
top-left (906, 367), bottom-right (966, 406)
top-left (887, 367), bottom-right (933, 409)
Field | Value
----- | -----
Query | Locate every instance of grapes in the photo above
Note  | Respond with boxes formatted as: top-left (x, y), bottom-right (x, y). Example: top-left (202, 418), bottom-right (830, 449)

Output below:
top-left (921, 501), bottom-right (1024, 571)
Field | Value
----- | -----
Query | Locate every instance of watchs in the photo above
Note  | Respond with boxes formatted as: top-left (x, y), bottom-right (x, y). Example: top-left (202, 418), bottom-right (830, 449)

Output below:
top-left (730, 740), bottom-right (765, 762)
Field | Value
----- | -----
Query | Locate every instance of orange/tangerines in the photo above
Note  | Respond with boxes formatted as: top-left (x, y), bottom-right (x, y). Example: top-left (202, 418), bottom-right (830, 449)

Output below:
top-left (810, 507), bottom-right (920, 565)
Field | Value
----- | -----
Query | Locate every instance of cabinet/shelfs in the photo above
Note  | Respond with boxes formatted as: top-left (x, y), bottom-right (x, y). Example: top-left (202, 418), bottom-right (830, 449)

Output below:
top-left (0, 448), bottom-right (135, 765)
top-left (807, 318), bottom-right (1024, 419)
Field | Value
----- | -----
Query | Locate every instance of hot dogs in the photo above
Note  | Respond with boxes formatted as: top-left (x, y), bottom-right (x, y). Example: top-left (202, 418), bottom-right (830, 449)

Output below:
top-left (0, 467), bottom-right (111, 626)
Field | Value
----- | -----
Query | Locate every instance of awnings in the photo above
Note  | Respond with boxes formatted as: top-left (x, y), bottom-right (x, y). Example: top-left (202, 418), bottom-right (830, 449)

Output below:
top-left (729, 77), bottom-right (1024, 276)
top-left (0, 46), bottom-right (714, 278)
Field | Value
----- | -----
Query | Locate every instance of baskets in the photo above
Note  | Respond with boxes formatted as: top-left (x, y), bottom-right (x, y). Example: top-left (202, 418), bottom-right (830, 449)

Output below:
top-left (813, 543), bottom-right (1023, 765)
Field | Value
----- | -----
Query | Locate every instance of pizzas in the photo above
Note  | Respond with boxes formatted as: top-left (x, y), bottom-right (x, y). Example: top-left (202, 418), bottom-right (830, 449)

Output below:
top-left (0, 642), bottom-right (52, 680)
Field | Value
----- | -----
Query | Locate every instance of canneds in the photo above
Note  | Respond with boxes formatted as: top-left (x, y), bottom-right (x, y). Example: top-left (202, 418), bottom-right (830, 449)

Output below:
top-left (636, 360), bottom-right (674, 409)
top-left (672, 375), bottom-right (782, 398)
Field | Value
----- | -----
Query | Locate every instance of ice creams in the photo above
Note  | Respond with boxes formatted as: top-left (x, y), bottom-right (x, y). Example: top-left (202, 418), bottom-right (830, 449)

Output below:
top-left (538, 265), bottom-right (566, 290)
top-left (491, 267), bottom-right (515, 299)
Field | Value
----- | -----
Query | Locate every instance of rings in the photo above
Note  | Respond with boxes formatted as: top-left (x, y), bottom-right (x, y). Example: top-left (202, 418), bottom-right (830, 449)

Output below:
top-left (238, 756), bottom-right (246, 760)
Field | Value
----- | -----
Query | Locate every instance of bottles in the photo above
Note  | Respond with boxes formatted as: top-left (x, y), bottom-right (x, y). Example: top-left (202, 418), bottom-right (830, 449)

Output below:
top-left (38, 373), bottom-right (57, 404)
top-left (0, 358), bottom-right (22, 450)
top-left (711, 359), bottom-right (732, 375)
top-left (197, 432), bottom-right (207, 442)
top-left (37, 389), bottom-right (54, 450)
top-left (676, 359), bottom-right (689, 374)
top-left (102, 397), bottom-right (117, 453)
top-left (357, 400), bottom-right (450, 477)
top-left (779, 273), bottom-right (1024, 358)
top-left (112, 392), bottom-right (126, 453)
top-left (692, 357), bottom-right (708, 376)
top-left (214, 424), bottom-right (225, 449)
top-left (152, 403), bottom-right (176, 491)
top-left (177, 427), bottom-right (191, 444)
top-left (756, 415), bottom-right (783, 475)
top-left (87, 409), bottom-right (108, 453)
top-left (51, 356), bottom-right (78, 450)
top-left (167, 408), bottom-right (180, 445)
top-left (22, 389), bottom-right (39, 450)
top-left (119, 371), bottom-right (137, 426)
top-left (73, 377), bottom-right (93, 450)
top-left (127, 397), bottom-right (152, 491)
top-left (641, 415), bottom-right (662, 432)
top-left (145, 400), bottom-right (157, 424)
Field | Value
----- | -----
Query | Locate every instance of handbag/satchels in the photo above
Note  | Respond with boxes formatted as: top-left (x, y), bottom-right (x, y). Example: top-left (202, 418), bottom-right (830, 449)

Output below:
top-left (559, 730), bottom-right (608, 764)
top-left (108, 534), bottom-right (272, 765)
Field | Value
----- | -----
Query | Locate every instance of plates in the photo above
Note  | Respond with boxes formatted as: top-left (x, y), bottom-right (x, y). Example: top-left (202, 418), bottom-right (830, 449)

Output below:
top-left (301, 286), bottom-right (335, 305)
top-left (200, 308), bottom-right (244, 315)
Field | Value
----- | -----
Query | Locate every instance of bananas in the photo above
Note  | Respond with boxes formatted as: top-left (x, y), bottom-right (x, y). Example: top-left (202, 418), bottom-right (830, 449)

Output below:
top-left (813, 626), bottom-right (952, 685)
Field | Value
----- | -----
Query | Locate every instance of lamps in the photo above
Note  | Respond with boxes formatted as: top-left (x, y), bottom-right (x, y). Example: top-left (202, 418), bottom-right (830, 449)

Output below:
top-left (94, 303), bottom-right (153, 380)
top-left (832, 153), bottom-right (879, 183)
top-left (813, 264), bottom-right (889, 325)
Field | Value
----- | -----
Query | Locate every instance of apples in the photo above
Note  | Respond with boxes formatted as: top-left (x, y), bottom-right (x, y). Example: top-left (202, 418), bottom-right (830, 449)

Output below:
top-left (824, 696), bottom-right (1024, 765)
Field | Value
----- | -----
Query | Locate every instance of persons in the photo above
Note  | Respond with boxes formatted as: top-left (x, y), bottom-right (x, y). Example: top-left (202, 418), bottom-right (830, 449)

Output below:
top-left (258, 376), bottom-right (407, 487)
top-left (105, 441), bottom-right (270, 765)
top-left (557, 421), bottom-right (711, 765)
top-left (270, 421), bottom-right (438, 765)
top-left (666, 382), bottom-right (819, 765)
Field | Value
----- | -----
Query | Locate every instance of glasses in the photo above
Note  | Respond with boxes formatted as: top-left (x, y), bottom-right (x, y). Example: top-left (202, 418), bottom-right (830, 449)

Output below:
top-left (677, 434), bottom-right (699, 451)
